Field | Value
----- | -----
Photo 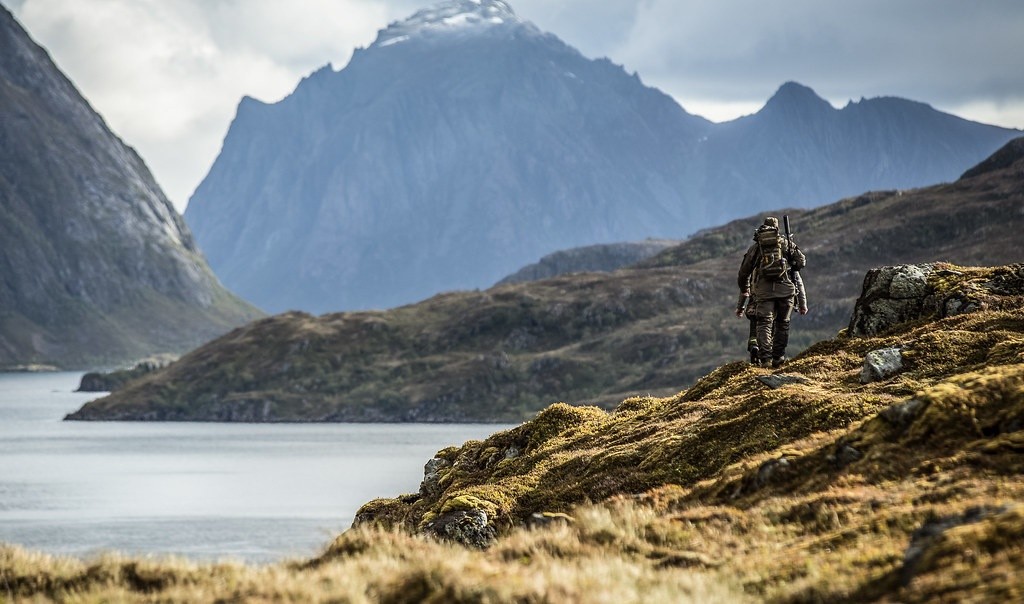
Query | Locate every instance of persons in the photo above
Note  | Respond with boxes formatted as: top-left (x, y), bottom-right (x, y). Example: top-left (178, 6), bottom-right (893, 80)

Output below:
top-left (736, 217), bottom-right (808, 370)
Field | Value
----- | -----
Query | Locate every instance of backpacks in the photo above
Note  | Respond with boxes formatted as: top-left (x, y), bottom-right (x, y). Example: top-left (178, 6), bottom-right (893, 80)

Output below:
top-left (750, 239), bottom-right (796, 301)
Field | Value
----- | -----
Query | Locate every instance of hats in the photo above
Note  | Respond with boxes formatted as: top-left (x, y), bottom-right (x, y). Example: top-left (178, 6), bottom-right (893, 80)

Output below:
top-left (753, 225), bottom-right (778, 241)
top-left (764, 216), bottom-right (778, 229)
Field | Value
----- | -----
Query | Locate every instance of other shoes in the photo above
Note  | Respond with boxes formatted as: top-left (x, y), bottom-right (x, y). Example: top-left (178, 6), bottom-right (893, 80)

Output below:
top-left (762, 360), bottom-right (772, 369)
top-left (772, 355), bottom-right (785, 368)
top-left (750, 345), bottom-right (760, 365)
